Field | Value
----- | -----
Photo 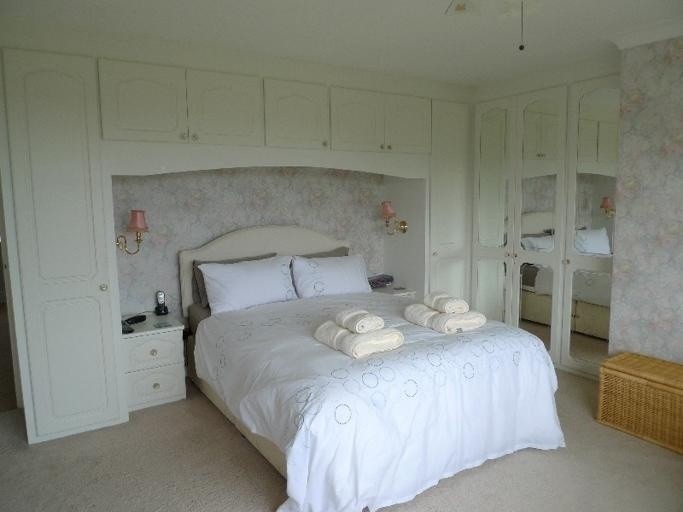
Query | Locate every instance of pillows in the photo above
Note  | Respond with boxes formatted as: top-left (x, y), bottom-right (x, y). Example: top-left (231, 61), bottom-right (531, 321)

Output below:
top-left (191, 246), bottom-right (374, 315)
top-left (575, 228), bottom-right (612, 257)
top-left (522, 236), bottom-right (555, 253)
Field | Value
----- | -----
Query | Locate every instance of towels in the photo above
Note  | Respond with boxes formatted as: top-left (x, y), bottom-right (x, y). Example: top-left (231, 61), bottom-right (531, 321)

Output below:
top-left (315, 307), bottom-right (405, 357)
top-left (404, 289), bottom-right (489, 334)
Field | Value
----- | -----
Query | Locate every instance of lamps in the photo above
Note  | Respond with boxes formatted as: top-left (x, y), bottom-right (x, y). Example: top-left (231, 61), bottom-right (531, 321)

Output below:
top-left (116, 210), bottom-right (149, 255)
top-left (600, 196), bottom-right (616, 220)
top-left (380, 200), bottom-right (409, 237)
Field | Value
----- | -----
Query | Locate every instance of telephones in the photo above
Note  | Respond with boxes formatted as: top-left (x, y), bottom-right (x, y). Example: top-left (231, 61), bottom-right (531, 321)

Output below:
top-left (154, 291), bottom-right (168, 316)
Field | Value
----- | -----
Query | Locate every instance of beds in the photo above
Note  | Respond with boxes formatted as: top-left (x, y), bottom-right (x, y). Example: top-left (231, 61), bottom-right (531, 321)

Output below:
top-left (524, 213), bottom-right (558, 236)
top-left (178, 224), bottom-right (567, 511)
top-left (522, 262), bottom-right (552, 328)
top-left (575, 211), bottom-right (594, 230)
top-left (574, 271), bottom-right (610, 342)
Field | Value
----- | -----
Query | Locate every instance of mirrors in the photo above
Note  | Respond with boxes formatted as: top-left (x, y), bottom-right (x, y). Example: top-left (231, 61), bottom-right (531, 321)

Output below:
top-left (481, 107), bottom-right (511, 247)
top-left (520, 263), bottom-right (554, 351)
top-left (573, 86), bottom-right (615, 256)
top-left (573, 270), bottom-right (611, 364)
top-left (521, 95), bottom-right (558, 253)
top-left (478, 256), bottom-right (506, 325)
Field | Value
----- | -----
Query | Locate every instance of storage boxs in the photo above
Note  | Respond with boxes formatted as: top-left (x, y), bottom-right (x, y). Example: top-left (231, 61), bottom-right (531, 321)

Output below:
top-left (595, 351), bottom-right (683, 455)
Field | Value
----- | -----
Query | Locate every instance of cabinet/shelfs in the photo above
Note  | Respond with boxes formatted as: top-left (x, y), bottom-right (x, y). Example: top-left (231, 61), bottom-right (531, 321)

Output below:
top-left (95, 30), bottom-right (432, 180)
top-left (383, 76), bottom-right (477, 310)
top-left (122, 310), bottom-right (186, 413)
top-left (569, 74), bottom-right (617, 380)
top-left (0, 20), bottom-right (130, 448)
top-left (476, 84), bottom-right (566, 375)
